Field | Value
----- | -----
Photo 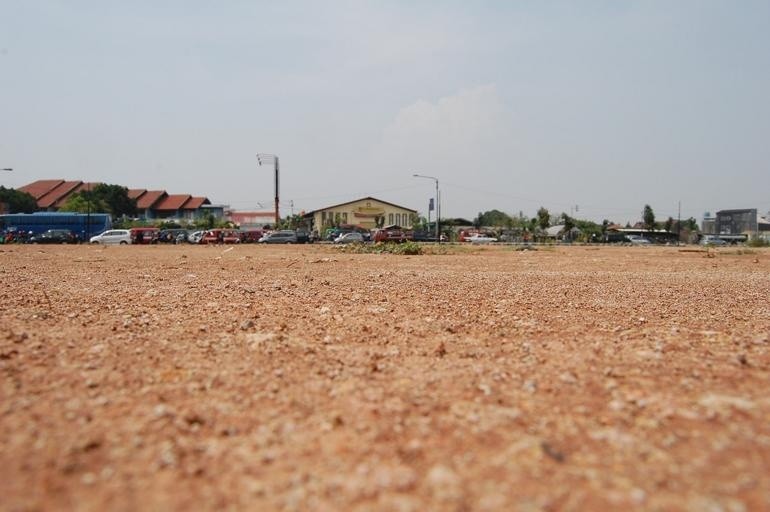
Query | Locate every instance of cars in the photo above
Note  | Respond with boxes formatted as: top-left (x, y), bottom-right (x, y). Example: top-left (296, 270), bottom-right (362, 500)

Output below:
top-left (153, 227), bottom-right (189, 244)
top-left (258, 231), bottom-right (297, 242)
top-left (298, 230), bottom-right (313, 242)
top-left (88, 229), bottom-right (132, 244)
top-left (464, 233), bottom-right (498, 243)
top-left (324, 226), bottom-right (405, 245)
top-left (625, 234), bottom-right (652, 245)
top-left (28, 228), bottom-right (75, 241)
top-left (131, 227), bottom-right (160, 243)
top-left (189, 227), bottom-right (262, 245)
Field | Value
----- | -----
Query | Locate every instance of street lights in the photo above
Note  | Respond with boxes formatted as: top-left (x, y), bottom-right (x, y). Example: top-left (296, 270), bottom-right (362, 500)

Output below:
top-left (413, 173), bottom-right (442, 241)
top-left (0, 167), bottom-right (14, 171)
top-left (256, 152), bottom-right (281, 231)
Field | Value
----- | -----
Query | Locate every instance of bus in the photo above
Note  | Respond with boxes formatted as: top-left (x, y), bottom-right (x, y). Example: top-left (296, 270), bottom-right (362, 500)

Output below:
top-left (1, 210), bottom-right (114, 243)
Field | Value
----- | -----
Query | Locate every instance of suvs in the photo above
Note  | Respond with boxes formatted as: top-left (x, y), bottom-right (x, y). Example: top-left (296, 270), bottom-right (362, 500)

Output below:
top-left (700, 234), bottom-right (730, 246)
